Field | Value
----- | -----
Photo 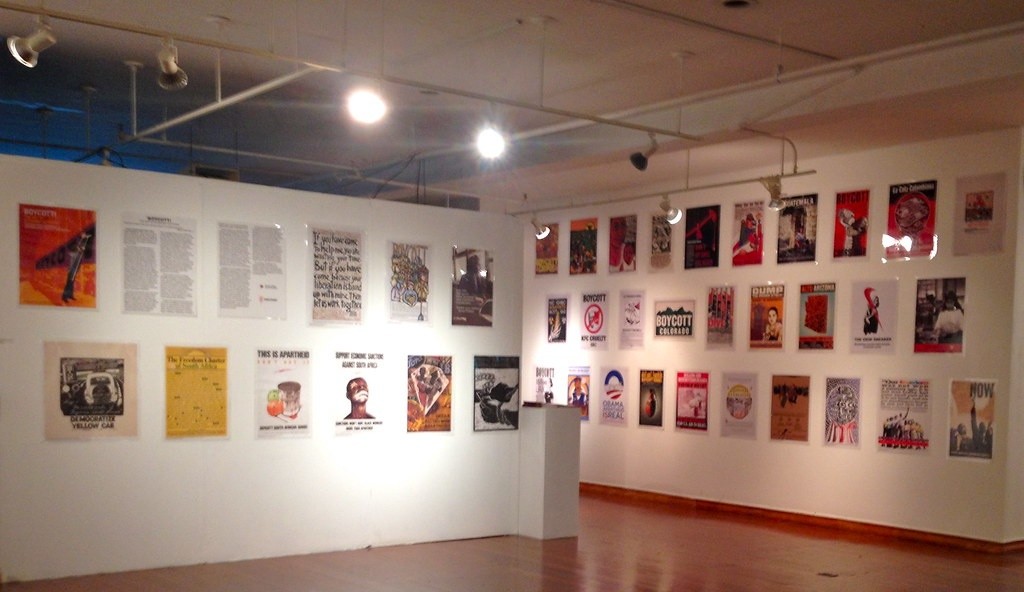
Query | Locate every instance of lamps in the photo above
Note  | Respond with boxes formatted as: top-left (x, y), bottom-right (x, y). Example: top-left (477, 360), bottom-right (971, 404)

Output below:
top-left (6, 12), bottom-right (56, 68)
top-left (157, 37), bottom-right (188, 90)
top-left (630, 132), bottom-right (659, 171)
top-left (660, 195), bottom-right (682, 224)
top-left (764, 177), bottom-right (786, 211)
top-left (530, 213), bottom-right (550, 240)
